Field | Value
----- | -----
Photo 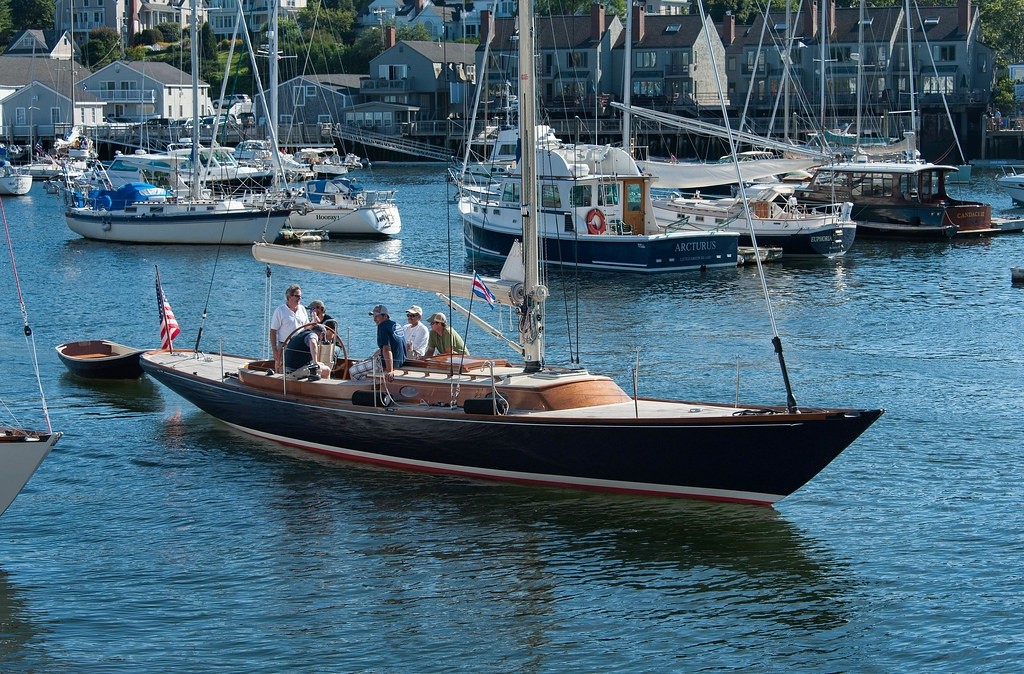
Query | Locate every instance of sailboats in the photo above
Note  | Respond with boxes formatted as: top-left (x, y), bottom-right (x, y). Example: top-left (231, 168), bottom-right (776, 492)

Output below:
top-left (138, 0), bottom-right (887, 506)
top-left (446, 0), bottom-right (1024, 274)
top-left (63, 0), bottom-right (293, 245)
top-left (227, 0), bottom-right (407, 236)
top-left (0, 0), bottom-right (373, 197)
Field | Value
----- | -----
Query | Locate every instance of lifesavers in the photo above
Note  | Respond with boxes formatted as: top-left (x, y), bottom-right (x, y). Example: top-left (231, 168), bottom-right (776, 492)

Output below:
top-left (76, 140), bottom-right (80, 147)
top-left (587, 208), bottom-right (606, 235)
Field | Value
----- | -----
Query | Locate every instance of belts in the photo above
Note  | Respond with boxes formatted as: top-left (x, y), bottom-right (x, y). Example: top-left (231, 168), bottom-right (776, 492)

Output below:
top-left (286, 372), bottom-right (292, 374)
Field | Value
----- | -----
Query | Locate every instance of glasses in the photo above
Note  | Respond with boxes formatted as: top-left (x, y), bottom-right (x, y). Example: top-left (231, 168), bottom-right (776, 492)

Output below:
top-left (406, 314), bottom-right (418, 317)
top-left (309, 307), bottom-right (320, 311)
top-left (293, 294), bottom-right (302, 298)
top-left (430, 322), bottom-right (439, 325)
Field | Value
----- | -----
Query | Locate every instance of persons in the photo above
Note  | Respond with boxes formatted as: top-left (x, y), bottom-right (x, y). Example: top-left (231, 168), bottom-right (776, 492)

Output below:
top-left (995, 108), bottom-right (1001, 130)
top-left (270, 285), bottom-right (320, 373)
top-left (987, 107), bottom-right (993, 130)
top-left (285, 324), bottom-right (331, 380)
top-left (306, 300), bottom-right (342, 378)
top-left (425, 313), bottom-right (470, 356)
top-left (403, 305), bottom-right (429, 359)
top-left (349, 304), bottom-right (407, 382)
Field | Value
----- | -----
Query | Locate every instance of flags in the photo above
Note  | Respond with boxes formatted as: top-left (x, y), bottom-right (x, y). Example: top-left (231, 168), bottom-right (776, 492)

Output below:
top-left (156, 276), bottom-right (181, 349)
top-left (472, 272), bottom-right (495, 310)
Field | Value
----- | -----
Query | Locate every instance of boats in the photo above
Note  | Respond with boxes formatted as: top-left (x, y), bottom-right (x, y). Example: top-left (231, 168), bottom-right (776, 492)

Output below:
top-left (1, 197), bottom-right (66, 520)
top-left (54, 339), bottom-right (156, 380)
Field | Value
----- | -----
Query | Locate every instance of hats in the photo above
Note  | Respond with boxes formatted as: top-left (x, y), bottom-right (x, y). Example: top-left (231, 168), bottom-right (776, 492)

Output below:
top-left (306, 300), bottom-right (324, 310)
top-left (405, 305), bottom-right (422, 318)
top-left (369, 305), bottom-right (389, 316)
top-left (320, 314), bottom-right (336, 330)
top-left (426, 312), bottom-right (446, 323)
